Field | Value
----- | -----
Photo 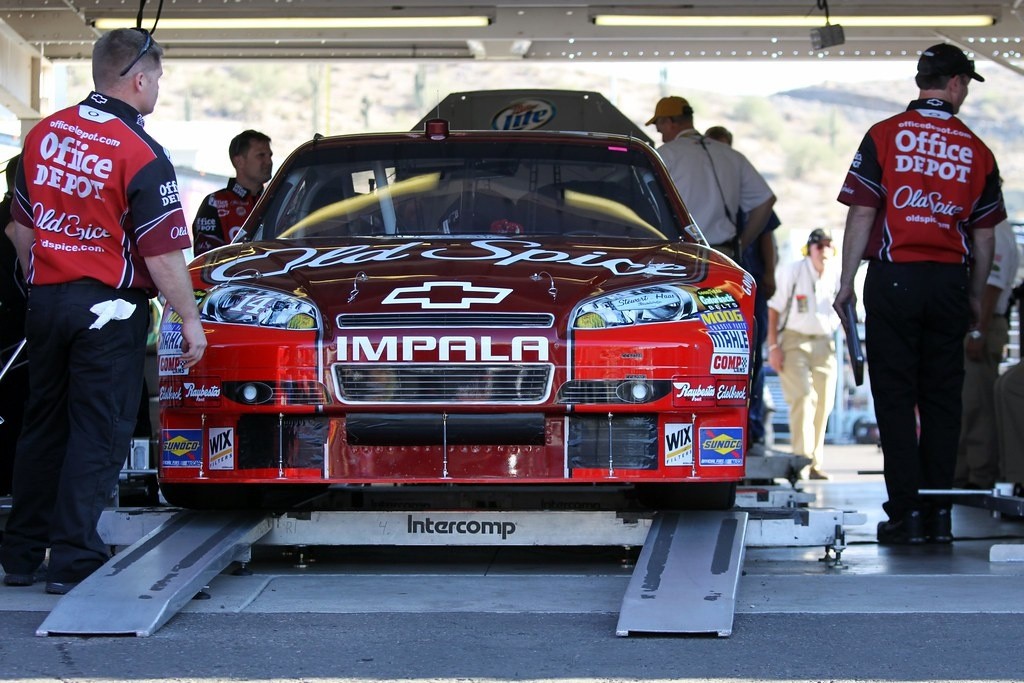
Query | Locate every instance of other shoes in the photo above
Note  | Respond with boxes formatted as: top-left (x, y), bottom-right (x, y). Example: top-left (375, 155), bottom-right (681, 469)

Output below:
top-left (44, 547), bottom-right (101, 595)
top-left (1, 542), bottom-right (43, 588)
top-left (799, 466), bottom-right (828, 479)
top-left (877, 510), bottom-right (927, 545)
top-left (927, 506), bottom-right (955, 544)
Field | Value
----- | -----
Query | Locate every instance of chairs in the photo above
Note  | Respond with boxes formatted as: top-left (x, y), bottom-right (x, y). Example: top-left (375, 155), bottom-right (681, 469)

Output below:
top-left (514, 179), bottom-right (661, 238)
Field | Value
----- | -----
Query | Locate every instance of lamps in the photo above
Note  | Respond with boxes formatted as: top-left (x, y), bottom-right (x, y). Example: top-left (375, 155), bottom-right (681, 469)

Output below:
top-left (83, 6), bottom-right (497, 29)
top-left (810, 0), bottom-right (845, 50)
top-left (588, 6), bottom-right (1000, 27)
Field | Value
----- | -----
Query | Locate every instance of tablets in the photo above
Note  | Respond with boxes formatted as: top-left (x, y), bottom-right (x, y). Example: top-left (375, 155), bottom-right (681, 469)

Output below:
top-left (844, 302), bottom-right (865, 386)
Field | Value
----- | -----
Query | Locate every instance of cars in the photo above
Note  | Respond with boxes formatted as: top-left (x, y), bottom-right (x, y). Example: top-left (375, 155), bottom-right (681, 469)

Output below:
top-left (155, 117), bottom-right (757, 512)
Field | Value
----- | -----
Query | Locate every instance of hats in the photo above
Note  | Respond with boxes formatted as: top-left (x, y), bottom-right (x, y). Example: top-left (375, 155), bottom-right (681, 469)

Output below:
top-left (918, 42), bottom-right (985, 82)
top-left (807, 228), bottom-right (832, 245)
top-left (645, 96), bottom-right (692, 126)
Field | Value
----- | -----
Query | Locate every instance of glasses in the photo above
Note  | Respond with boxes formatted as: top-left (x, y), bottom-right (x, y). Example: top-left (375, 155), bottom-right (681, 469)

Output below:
top-left (119, 27), bottom-right (154, 76)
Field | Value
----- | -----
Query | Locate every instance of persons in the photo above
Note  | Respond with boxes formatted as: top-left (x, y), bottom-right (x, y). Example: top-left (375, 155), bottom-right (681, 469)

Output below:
top-left (955, 222), bottom-right (1021, 490)
top-left (0, 28), bottom-right (207, 593)
top-left (193, 130), bottom-right (272, 256)
top-left (646, 97), bottom-right (777, 263)
top-left (704, 126), bottom-right (781, 450)
top-left (832, 43), bottom-right (1007, 543)
top-left (767, 229), bottom-right (840, 481)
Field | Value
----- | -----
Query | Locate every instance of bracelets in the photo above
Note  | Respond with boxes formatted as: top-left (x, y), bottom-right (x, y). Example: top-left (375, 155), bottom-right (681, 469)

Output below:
top-left (768, 344), bottom-right (777, 351)
top-left (842, 270), bottom-right (856, 276)
